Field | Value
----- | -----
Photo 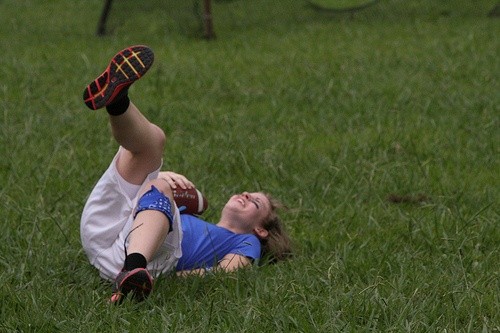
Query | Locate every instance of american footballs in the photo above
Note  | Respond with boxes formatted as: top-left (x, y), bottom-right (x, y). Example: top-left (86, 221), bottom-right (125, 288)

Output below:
top-left (171, 182), bottom-right (208, 216)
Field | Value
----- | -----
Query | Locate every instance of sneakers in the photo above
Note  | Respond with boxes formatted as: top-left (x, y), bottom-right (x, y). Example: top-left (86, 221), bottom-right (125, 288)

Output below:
top-left (83, 44), bottom-right (154, 111)
top-left (111, 268), bottom-right (153, 305)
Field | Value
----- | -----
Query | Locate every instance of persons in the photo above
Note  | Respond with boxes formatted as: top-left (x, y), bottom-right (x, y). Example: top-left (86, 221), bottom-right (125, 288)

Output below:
top-left (79, 46), bottom-right (290, 305)
